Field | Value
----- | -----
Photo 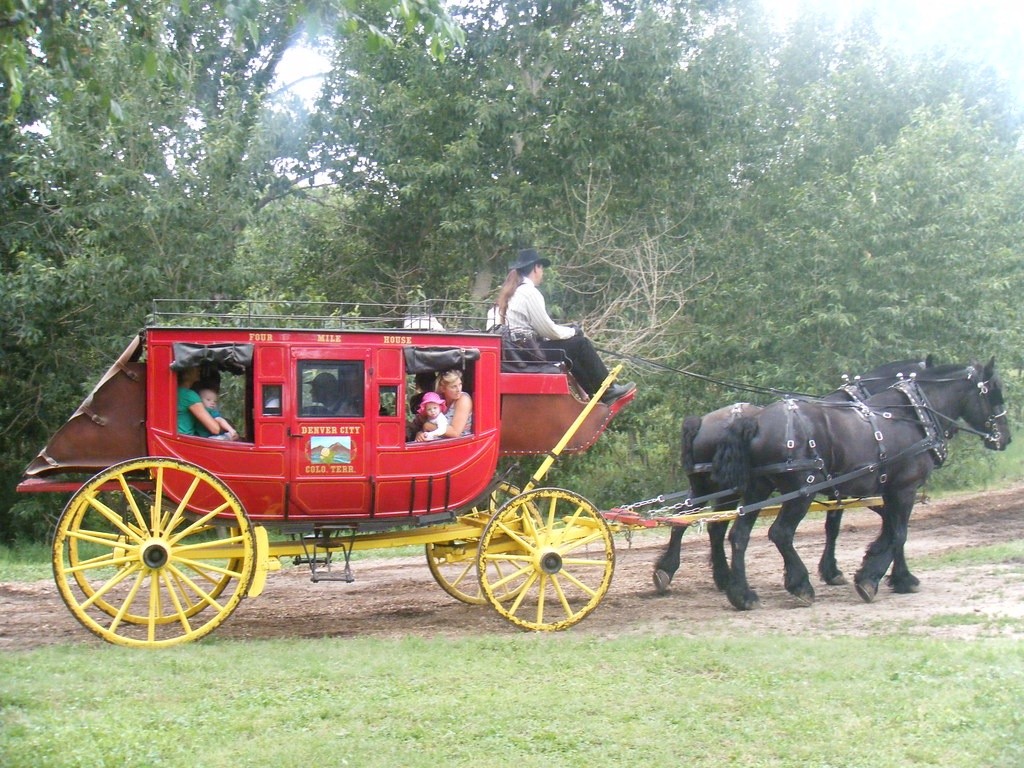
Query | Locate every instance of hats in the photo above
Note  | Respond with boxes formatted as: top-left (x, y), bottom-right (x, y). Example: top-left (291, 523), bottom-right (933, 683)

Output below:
top-left (413, 373), bottom-right (436, 383)
top-left (509, 248), bottom-right (551, 268)
top-left (304, 372), bottom-right (337, 387)
top-left (417, 392), bottom-right (446, 414)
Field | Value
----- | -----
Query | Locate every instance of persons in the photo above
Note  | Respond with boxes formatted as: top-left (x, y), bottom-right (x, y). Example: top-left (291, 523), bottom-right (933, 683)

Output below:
top-left (406, 369), bottom-right (473, 442)
top-left (177, 363), bottom-right (241, 441)
top-left (486, 269), bottom-right (590, 402)
top-left (504, 248), bottom-right (636, 408)
top-left (305, 373), bottom-right (358, 414)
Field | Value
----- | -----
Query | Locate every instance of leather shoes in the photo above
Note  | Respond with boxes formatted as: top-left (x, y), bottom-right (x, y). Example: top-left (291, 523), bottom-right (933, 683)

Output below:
top-left (603, 381), bottom-right (636, 405)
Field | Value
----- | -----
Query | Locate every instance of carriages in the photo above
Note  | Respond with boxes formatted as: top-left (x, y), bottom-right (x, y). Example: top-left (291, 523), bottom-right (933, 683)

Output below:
top-left (15, 301), bottom-right (1014, 653)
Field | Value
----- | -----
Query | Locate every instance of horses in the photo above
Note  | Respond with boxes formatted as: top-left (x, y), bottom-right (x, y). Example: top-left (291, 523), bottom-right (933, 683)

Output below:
top-left (651, 352), bottom-right (1012, 610)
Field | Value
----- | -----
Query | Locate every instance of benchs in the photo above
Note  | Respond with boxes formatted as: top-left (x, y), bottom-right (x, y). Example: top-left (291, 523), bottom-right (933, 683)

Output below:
top-left (500, 323), bottom-right (636, 455)
